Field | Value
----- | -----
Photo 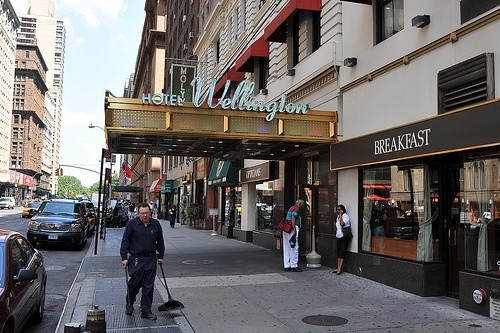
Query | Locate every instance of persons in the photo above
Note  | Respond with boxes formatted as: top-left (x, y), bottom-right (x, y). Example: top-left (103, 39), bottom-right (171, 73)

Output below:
top-left (120, 203), bottom-right (165, 320)
top-left (331, 204), bottom-right (351, 274)
top-left (282, 200), bottom-right (305, 272)
top-left (129, 201), bottom-right (176, 228)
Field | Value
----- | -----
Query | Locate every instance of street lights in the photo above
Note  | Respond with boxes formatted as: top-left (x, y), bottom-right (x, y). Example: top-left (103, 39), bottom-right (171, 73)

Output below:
top-left (30, 171), bottom-right (43, 202)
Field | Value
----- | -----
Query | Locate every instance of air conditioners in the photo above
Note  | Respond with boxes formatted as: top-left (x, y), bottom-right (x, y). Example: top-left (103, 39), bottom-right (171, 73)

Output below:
top-left (183, 44), bottom-right (188, 50)
top-left (189, 32), bottom-right (194, 39)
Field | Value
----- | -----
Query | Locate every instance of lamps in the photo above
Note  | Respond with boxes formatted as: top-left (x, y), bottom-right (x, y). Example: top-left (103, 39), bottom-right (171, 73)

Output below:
top-left (344, 57), bottom-right (357, 67)
top-left (286, 69), bottom-right (295, 76)
top-left (260, 89), bottom-right (268, 95)
top-left (412, 15), bottom-right (430, 28)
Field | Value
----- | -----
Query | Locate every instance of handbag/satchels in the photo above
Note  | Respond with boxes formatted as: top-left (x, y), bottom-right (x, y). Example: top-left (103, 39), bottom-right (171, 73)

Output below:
top-left (278, 217), bottom-right (292, 233)
top-left (342, 227), bottom-right (352, 238)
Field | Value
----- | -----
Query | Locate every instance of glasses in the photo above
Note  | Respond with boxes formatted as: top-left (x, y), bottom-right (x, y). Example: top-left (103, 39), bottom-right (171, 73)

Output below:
top-left (139, 212), bottom-right (149, 215)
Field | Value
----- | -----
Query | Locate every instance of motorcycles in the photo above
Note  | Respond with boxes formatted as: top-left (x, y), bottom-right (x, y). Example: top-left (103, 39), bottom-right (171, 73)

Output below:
top-left (105, 200), bottom-right (129, 227)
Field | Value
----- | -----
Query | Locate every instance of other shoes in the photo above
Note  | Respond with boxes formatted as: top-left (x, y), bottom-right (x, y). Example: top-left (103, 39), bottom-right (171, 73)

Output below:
top-left (337, 271), bottom-right (342, 275)
top-left (171, 225), bottom-right (174, 228)
top-left (285, 268), bottom-right (291, 271)
top-left (291, 267), bottom-right (302, 272)
top-left (332, 270), bottom-right (337, 273)
top-left (125, 303), bottom-right (134, 314)
top-left (141, 310), bottom-right (157, 321)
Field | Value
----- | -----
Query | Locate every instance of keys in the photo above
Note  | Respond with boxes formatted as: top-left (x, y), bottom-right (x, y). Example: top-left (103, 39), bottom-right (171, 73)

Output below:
top-left (135, 262), bottom-right (138, 266)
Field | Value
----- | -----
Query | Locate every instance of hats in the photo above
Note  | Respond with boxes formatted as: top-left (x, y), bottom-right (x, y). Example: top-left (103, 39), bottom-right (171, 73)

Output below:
top-left (289, 235), bottom-right (296, 248)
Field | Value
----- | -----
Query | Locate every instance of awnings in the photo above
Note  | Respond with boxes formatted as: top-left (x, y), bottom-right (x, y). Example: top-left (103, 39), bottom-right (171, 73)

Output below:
top-left (263, 0), bottom-right (322, 43)
top-left (41, 191), bottom-right (52, 197)
top-left (236, 34), bottom-right (269, 73)
top-left (149, 178), bottom-right (165, 192)
top-left (208, 158), bottom-right (238, 186)
top-left (212, 65), bottom-right (245, 98)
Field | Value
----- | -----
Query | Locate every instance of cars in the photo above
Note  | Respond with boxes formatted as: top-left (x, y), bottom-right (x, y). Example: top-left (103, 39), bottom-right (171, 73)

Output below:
top-left (22, 197), bottom-right (45, 218)
top-left (0, 197), bottom-right (16, 210)
top-left (0, 227), bottom-right (50, 333)
top-left (26, 198), bottom-right (96, 250)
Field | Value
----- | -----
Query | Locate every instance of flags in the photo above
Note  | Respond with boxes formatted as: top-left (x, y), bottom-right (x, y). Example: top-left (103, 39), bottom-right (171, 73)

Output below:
top-left (124, 166), bottom-right (134, 178)
